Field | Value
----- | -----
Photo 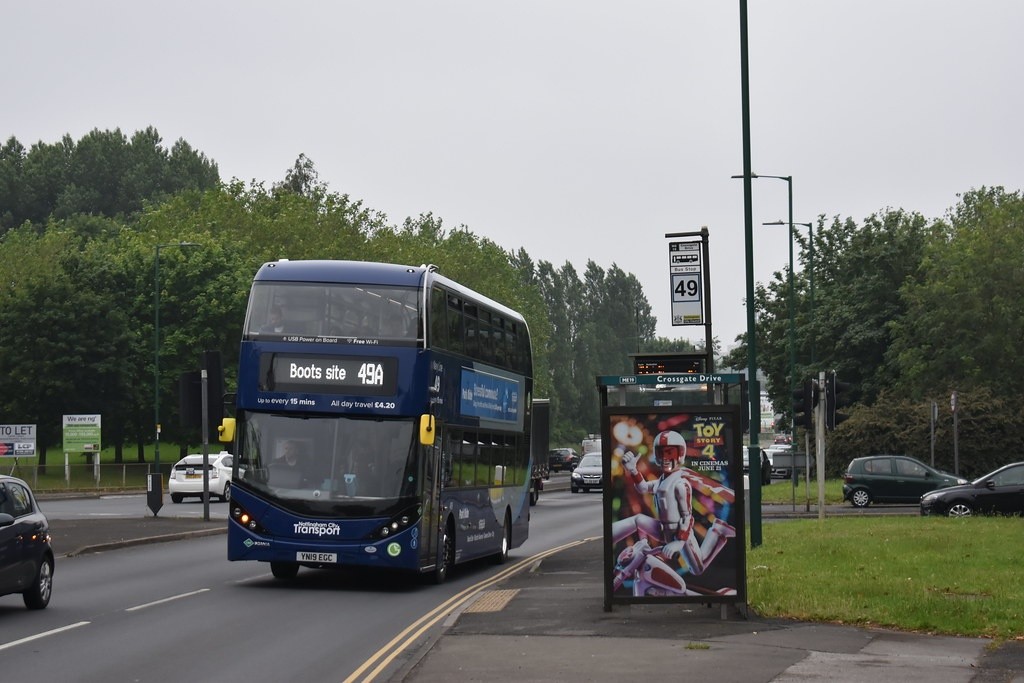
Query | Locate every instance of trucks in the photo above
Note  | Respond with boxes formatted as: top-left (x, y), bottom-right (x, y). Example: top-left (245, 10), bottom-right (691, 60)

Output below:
top-left (530, 399), bottom-right (551, 507)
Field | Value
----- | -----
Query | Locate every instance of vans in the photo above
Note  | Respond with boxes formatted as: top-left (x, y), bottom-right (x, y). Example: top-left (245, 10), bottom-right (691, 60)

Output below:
top-left (582, 439), bottom-right (601, 455)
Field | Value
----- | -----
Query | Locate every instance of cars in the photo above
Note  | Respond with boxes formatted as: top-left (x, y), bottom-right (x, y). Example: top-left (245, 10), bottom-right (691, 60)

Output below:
top-left (570, 453), bottom-right (603, 493)
top-left (920, 462), bottom-right (1024, 517)
top-left (549, 448), bottom-right (580, 472)
top-left (168, 453), bottom-right (245, 503)
top-left (0, 474), bottom-right (54, 610)
top-left (742, 446), bottom-right (771, 486)
top-left (842, 455), bottom-right (968, 507)
top-left (763, 444), bottom-right (793, 479)
top-left (775, 434), bottom-right (791, 445)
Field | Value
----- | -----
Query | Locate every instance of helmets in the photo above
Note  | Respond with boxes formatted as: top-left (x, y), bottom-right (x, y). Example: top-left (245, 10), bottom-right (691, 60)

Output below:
top-left (652, 431), bottom-right (686, 466)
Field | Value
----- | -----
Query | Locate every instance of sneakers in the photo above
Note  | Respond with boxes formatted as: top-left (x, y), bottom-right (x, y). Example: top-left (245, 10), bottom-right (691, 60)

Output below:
top-left (712, 517), bottom-right (737, 538)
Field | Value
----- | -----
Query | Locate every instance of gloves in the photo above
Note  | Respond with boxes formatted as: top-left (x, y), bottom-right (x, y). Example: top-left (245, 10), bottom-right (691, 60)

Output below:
top-left (622, 450), bottom-right (642, 475)
top-left (662, 540), bottom-right (685, 559)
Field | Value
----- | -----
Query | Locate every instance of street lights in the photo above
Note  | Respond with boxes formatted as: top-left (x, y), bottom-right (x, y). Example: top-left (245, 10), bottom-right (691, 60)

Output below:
top-left (153, 244), bottom-right (204, 463)
top-left (731, 174), bottom-right (799, 486)
top-left (763, 222), bottom-right (816, 368)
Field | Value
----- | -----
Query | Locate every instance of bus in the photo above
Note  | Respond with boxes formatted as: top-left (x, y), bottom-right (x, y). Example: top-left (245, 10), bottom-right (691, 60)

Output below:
top-left (217, 260), bottom-right (536, 578)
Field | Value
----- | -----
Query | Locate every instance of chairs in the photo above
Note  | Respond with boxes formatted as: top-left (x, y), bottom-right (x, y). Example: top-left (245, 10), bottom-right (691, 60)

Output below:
top-left (406, 317), bottom-right (423, 339)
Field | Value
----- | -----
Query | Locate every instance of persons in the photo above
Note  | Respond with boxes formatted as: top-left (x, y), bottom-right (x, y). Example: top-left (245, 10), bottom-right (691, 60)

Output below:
top-left (269, 440), bottom-right (317, 489)
top-left (260, 307), bottom-right (296, 333)
top-left (329, 325), bottom-right (341, 336)
